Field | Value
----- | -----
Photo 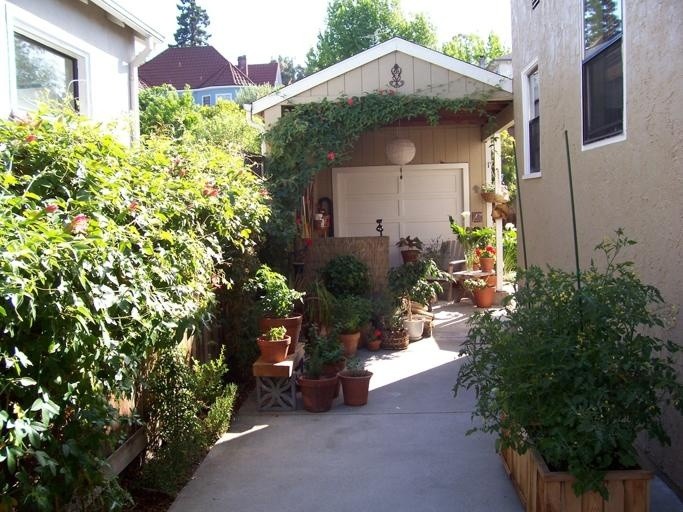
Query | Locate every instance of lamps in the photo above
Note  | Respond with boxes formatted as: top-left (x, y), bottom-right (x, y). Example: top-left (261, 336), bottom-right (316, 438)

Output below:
top-left (386, 119), bottom-right (417, 166)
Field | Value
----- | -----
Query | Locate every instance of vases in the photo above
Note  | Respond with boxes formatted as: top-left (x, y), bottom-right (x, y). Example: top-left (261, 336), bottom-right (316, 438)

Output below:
top-left (479, 257), bottom-right (495, 273)
top-left (368, 340), bottom-right (381, 351)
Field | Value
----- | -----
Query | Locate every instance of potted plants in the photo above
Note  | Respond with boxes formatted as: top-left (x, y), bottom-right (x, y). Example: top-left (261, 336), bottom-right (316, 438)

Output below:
top-left (249, 263), bottom-right (306, 355)
top-left (463, 279), bottom-right (495, 308)
top-left (450, 225), bottom-right (683, 512)
top-left (385, 258), bottom-right (458, 341)
top-left (256, 333), bottom-right (291, 363)
top-left (393, 235), bottom-right (426, 264)
top-left (300, 254), bottom-right (373, 413)
top-left (479, 184), bottom-right (496, 203)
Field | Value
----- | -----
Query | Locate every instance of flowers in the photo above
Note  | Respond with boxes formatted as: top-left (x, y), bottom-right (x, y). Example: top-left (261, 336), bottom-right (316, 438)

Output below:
top-left (477, 246), bottom-right (496, 257)
top-left (367, 328), bottom-right (382, 340)
